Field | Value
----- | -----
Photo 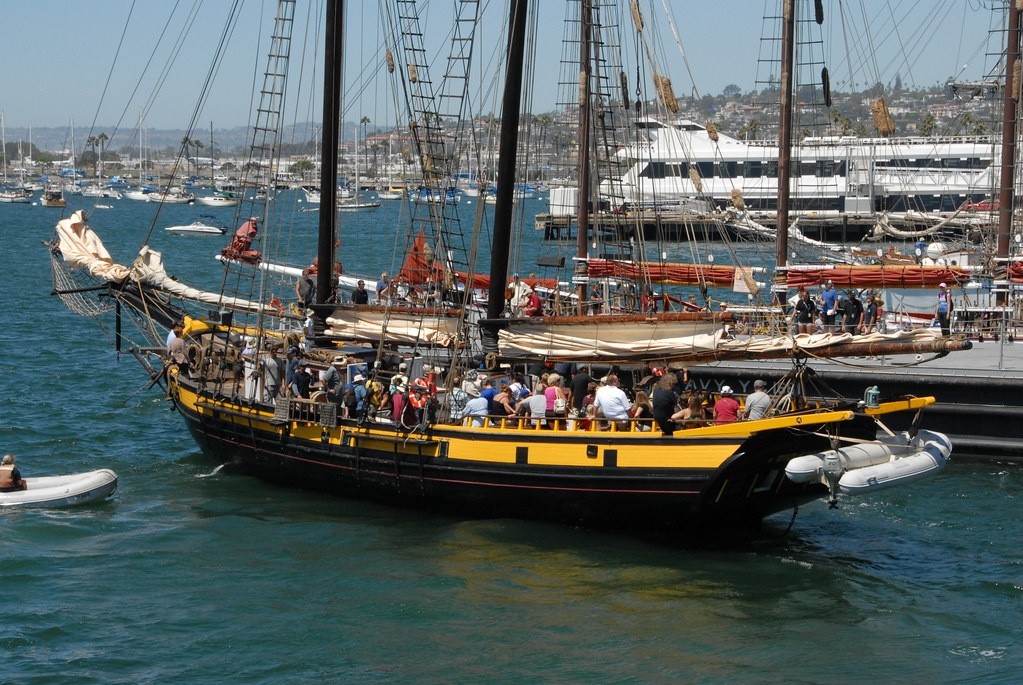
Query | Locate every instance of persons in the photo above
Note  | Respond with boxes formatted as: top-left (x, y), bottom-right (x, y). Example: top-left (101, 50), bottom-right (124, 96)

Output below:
top-left (682, 295), bottom-right (698, 313)
top-left (508, 273), bottom-right (543, 317)
top-left (788, 279), bottom-right (883, 335)
top-left (352, 280), bottom-right (369, 305)
top-left (263, 346), bottom-right (771, 432)
top-left (304, 309), bottom-right (313, 350)
top-left (590, 283), bottom-right (603, 314)
top-left (914, 238), bottom-right (928, 265)
top-left (452, 273), bottom-right (464, 292)
top-left (168, 321), bottom-right (193, 380)
top-left (720, 302), bottom-right (736, 324)
top-left (376, 273), bottom-right (438, 307)
top-left (936, 283), bottom-right (951, 338)
top-left (0, 454), bottom-right (27, 492)
top-left (295, 271), bottom-right (315, 315)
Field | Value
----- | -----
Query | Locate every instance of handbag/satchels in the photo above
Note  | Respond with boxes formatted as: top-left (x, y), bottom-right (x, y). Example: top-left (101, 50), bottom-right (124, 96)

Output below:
top-left (553, 399), bottom-right (565, 414)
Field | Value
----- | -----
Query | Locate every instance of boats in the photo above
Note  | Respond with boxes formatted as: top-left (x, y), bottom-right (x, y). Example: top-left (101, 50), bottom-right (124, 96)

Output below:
top-left (784, 431), bottom-right (953, 501)
top-left (164, 214), bottom-right (228, 238)
top-left (597, 116), bottom-right (1023, 238)
top-left (0, 468), bottom-right (119, 509)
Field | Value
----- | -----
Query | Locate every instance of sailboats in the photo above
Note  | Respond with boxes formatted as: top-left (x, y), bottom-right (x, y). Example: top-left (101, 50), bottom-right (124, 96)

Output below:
top-left (0, 107), bottom-right (241, 209)
top-left (44, 0), bottom-right (1023, 538)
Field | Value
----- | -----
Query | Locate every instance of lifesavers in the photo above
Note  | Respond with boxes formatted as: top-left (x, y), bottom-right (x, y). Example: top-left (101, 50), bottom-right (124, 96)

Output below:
top-left (269, 299), bottom-right (285, 313)
top-left (887, 246), bottom-right (895, 254)
top-left (291, 302), bottom-right (298, 315)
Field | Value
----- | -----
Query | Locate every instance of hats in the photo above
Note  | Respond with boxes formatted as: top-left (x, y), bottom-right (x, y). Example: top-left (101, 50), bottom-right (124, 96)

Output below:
top-left (754, 380), bottom-right (766, 387)
top-left (352, 374), bottom-right (366, 383)
top-left (298, 359), bottom-right (308, 368)
top-left (512, 272), bottom-right (519, 278)
top-left (848, 289), bottom-right (857, 295)
top-left (463, 369), bottom-right (477, 379)
top-left (721, 385), bottom-right (734, 393)
top-left (938, 283), bottom-right (946, 287)
top-left (465, 387), bottom-right (481, 397)
top-left (286, 347), bottom-right (298, 354)
top-left (331, 355), bottom-right (346, 366)
top-left (399, 362), bottom-right (407, 370)
